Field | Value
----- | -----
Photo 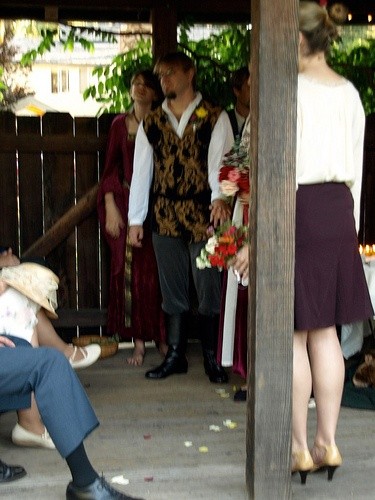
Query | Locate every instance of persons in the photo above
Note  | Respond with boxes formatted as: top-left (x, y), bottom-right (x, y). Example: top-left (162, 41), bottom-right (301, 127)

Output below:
top-left (207, 58), bottom-right (318, 408)
top-left (293, 3), bottom-right (375, 485)
top-left (0, 245), bottom-right (101, 450)
top-left (126, 46), bottom-right (227, 384)
top-left (94, 67), bottom-right (175, 366)
top-left (0, 332), bottom-right (147, 500)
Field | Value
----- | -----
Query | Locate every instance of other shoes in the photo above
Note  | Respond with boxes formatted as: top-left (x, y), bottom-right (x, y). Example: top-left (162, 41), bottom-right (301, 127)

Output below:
top-left (12, 422), bottom-right (57, 449)
top-left (68, 343), bottom-right (101, 369)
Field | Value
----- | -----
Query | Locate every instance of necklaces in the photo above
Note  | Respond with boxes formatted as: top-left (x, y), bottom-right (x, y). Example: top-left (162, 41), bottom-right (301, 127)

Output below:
top-left (132, 109), bottom-right (140, 124)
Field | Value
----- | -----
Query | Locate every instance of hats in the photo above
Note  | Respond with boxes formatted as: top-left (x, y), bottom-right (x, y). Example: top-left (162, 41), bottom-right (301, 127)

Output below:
top-left (0, 262), bottom-right (60, 319)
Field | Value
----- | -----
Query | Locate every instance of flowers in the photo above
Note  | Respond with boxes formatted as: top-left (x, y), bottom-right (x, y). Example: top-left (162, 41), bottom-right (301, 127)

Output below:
top-left (196, 224), bottom-right (249, 287)
top-left (217, 137), bottom-right (250, 197)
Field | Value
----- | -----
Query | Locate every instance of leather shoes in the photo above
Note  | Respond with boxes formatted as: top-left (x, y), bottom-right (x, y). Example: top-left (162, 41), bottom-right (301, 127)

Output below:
top-left (203, 355), bottom-right (229, 384)
top-left (145, 345), bottom-right (189, 380)
top-left (66, 471), bottom-right (144, 500)
top-left (0, 459), bottom-right (27, 482)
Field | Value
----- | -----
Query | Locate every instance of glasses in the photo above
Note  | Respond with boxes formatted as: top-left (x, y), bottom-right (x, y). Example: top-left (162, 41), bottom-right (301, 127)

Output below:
top-left (158, 69), bottom-right (176, 79)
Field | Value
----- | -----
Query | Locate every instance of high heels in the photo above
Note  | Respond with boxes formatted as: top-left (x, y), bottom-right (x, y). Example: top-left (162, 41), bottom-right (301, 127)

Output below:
top-left (291, 448), bottom-right (314, 485)
top-left (310, 445), bottom-right (342, 481)
